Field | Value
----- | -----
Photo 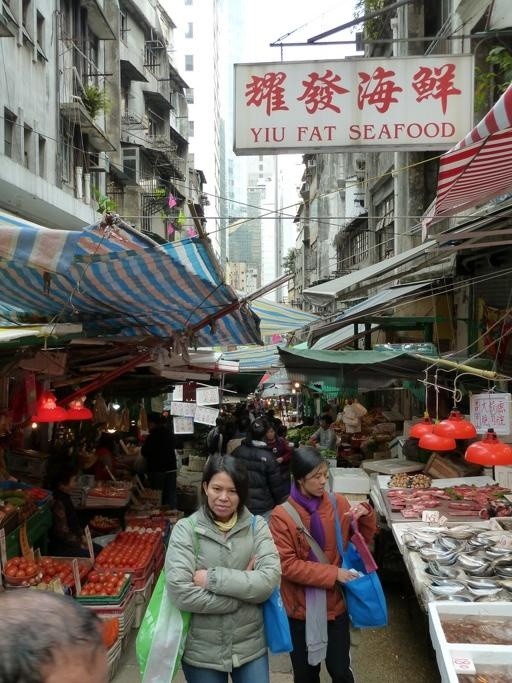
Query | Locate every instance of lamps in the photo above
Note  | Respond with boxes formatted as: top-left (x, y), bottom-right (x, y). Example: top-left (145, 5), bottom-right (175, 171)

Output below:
top-left (410, 378), bottom-right (512, 467)
top-left (30, 349), bottom-right (94, 423)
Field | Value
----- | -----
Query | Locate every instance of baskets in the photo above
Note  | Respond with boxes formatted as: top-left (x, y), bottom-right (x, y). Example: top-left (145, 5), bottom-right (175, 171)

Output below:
top-left (75, 573), bottom-right (136, 682)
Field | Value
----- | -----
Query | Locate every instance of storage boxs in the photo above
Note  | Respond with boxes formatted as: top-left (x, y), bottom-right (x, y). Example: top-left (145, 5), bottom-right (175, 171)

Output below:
top-left (387, 420), bottom-right (418, 461)
top-left (328, 467), bottom-right (371, 516)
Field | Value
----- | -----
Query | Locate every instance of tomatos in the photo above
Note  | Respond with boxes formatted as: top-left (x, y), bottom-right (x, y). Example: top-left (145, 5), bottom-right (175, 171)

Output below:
top-left (4, 555), bottom-right (90, 588)
top-left (93, 529), bottom-right (161, 567)
top-left (78, 569), bottom-right (128, 597)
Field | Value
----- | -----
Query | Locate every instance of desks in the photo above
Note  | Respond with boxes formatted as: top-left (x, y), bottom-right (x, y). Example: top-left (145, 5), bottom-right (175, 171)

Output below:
top-left (5, 496), bottom-right (55, 562)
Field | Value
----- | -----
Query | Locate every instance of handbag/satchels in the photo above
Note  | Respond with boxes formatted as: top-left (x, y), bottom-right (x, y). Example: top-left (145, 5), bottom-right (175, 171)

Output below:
top-left (263, 586), bottom-right (293, 653)
top-left (348, 510), bottom-right (378, 574)
top-left (136, 569), bottom-right (191, 683)
top-left (338, 542), bottom-right (387, 628)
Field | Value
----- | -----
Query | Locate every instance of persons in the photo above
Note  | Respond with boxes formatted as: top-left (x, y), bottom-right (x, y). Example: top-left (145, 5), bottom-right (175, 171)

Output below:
top-left (37, 465), bottom-right (103, 559)
top-left (0, 586), bottom-right (113, 683)
top-left (164, 452), bottom-right (282, 683)
top-left (269, 445), bottom-right (377, 683)
top-left (93, 393), bottom-right (367, 517)
